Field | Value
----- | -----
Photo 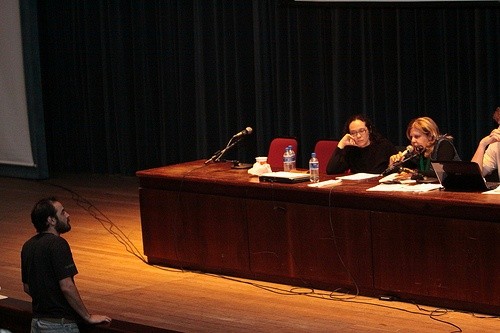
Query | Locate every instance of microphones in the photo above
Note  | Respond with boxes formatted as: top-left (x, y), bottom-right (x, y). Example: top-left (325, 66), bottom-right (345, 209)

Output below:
top-left (388, 145), bottom-right (414, 165)
top-left (233, 126), bottom-right (252, 138)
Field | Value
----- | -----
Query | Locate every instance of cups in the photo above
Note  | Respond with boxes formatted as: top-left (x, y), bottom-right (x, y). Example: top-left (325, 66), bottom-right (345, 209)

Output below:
top-left (255, 156), bottom-right (267, 165)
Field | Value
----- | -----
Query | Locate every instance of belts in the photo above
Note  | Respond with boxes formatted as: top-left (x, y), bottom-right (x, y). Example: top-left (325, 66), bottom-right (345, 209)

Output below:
top-left (39, 318), bottom-right (73, 324)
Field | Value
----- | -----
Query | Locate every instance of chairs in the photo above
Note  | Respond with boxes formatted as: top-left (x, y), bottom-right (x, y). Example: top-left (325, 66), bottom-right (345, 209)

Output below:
top-left (315, 140), bottom-right (351, 173)
top-left (265, 138), bottom-right (298, 167)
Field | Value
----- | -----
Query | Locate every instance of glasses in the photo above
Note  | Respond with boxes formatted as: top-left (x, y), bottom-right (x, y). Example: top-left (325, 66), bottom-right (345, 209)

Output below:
top-left (350, 128), bottom-right (367, 135)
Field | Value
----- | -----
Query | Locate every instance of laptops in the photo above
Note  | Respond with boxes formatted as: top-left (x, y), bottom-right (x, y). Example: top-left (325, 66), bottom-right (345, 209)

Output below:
top-left (431, 161), bottom-right (500, 191)
top-left (259, 171), bottom-right (310, 184)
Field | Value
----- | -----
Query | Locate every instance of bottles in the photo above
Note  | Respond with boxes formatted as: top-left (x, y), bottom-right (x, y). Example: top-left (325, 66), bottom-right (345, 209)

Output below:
top-left (288, 146), bottom-right (296, 171)
top-left (309, 153), bottom-right (319, 182)
top-left (283, 148), bottom-right (293, 172)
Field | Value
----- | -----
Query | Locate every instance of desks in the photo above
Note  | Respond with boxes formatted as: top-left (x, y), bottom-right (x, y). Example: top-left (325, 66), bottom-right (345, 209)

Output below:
top-left (135, 158), bottom-right (500, 315)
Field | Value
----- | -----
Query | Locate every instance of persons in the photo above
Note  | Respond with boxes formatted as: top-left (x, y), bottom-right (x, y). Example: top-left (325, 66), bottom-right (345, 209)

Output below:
top-left (388, 116), bottom-right (462, 180)
top-left (22, 196), bottom-right (112, 333)
top-left (325, 114), bottom-right (400, 176)
top-left (471, 106), bottom-right (500, 187)
